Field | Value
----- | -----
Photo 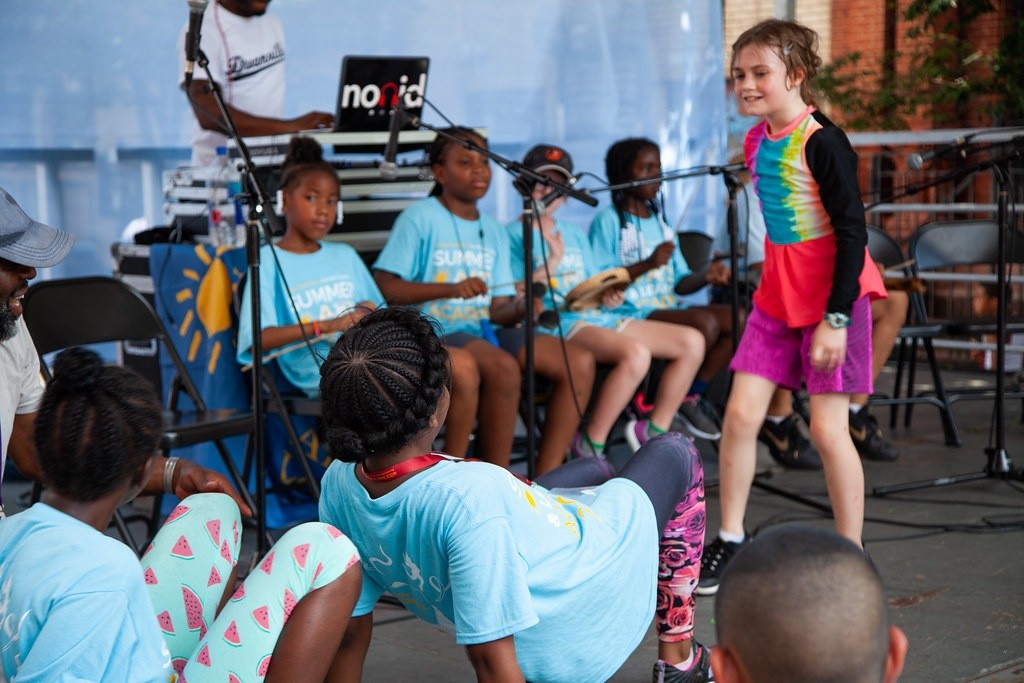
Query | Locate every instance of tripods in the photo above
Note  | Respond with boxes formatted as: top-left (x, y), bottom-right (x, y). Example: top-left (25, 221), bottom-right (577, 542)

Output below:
top-left (873, 140), bottom-right (1024, 495)
top-left (559, 161), bottom-right (836, 520)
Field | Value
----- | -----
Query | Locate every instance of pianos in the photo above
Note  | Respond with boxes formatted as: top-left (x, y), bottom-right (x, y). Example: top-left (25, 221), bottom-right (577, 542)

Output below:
top-left (162, 129), bottom-right (442, 215)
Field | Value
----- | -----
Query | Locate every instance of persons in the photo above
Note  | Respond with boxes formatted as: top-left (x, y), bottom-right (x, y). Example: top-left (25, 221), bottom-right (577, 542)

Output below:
top-left (237, 137), bottom-right (480, 459)
top-left (710, 523), bottom-right (907, 683)
top-left (718, 179), bottom-right (909, 469)
top-left (0, 369), bottom-right (363, 683)
top-left (178, 0), bottom-right (336, 191)
top-left (0, 185), bottom-right (252, 523)
top-left (316, 307), bottom-right (715, 683)
top-left (373, 127), bottom-right (731, 480)
top-left (697, 20), bottom-right (887, 594)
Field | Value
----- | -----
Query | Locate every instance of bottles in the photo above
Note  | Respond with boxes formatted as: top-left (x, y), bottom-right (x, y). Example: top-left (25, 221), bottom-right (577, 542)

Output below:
top-left (205, 146), bottom-right (247, 250)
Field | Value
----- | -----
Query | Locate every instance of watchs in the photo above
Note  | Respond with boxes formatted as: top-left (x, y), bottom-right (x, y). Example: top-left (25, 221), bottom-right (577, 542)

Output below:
top-left (824, 312), bottom-right (852, 328)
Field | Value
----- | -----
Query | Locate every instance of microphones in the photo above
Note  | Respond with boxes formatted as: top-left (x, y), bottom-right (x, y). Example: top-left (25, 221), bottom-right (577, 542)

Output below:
top-left (906, 133), bottom-right (974, 170)
top-left (529, 173), bottom-right (583, 217)
top-left (184, 0), bottom-right (208, 86)
top-left (379, 96), bottom-right (405, 180)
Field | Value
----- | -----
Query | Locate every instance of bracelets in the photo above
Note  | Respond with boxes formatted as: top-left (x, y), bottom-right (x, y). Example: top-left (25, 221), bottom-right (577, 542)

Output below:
top-left (163, 457), bottom-right (181, 495)
top-left (313, 319), bottom-right (321, 337)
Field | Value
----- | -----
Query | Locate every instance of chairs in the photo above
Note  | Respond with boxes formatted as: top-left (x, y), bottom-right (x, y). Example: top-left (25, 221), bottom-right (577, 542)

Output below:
top-left (15, 219), bottom-right (1023, 608)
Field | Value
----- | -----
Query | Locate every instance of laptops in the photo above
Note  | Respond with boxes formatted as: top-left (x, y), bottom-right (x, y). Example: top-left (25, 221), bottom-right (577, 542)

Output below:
top-left (300, 55), bottom-right (431, 133)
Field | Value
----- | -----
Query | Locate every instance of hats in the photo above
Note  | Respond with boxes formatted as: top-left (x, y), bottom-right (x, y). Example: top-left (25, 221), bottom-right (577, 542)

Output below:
top-left (0, 188), bottom-right (75, 268)
top-left (523, 143), bottom-right (573, 180)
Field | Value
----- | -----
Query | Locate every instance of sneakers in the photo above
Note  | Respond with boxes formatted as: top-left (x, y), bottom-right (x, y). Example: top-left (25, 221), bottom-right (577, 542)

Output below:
top-left (676, 396), bottom-right (721, 440)
top-left (626, 420), bottom-right (650, 453)
top-left (693, 528), bottom-right (752, 595)
top-left (848, 401), bottom-right (900, 463)
top-left (652, 637), bottom-right (714, 683)
top-left (861, 541), bottom-right (872, 561)
top-left (566, 432), bottom-right (606, 462)
top-left (757, 410), bottom-right (824, 472)
